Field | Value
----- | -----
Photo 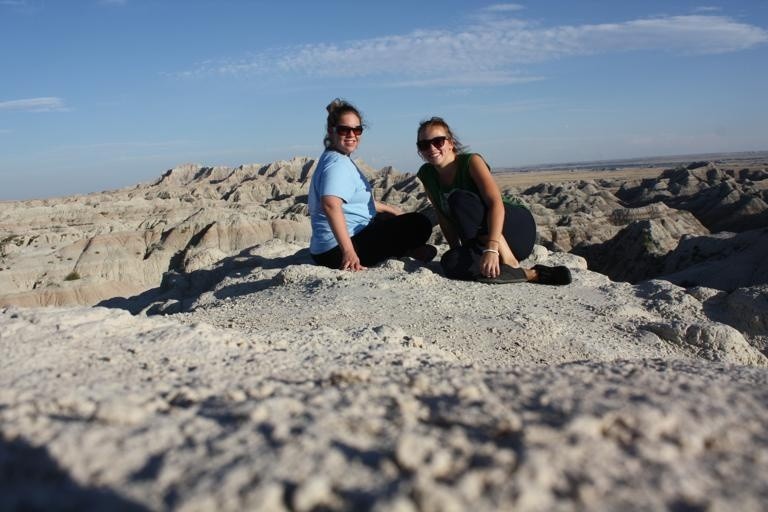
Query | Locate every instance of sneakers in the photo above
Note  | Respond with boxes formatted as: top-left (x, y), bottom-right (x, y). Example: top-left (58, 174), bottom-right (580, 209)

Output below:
top-left (531, 265), bottom-right (572, 284)
top-left (414, 244), bottom-right (436, 260)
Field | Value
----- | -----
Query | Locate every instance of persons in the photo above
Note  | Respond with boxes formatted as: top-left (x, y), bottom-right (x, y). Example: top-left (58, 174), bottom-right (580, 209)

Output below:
top-left (417, 117), bottom-right (572, 284)
top-left (308, 98), bottom-right (437, 272)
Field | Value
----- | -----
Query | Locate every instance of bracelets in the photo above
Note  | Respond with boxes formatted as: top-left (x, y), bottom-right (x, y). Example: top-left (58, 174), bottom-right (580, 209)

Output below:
top-left (487, 239), bottom-right (499, 243)
top-left (483, 249), bottom-right (499, 255)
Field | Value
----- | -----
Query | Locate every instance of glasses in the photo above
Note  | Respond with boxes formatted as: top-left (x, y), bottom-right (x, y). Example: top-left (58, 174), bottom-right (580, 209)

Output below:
top-left (417, 136), bottom-right (445, 150)
top-left (332, 125), bottom-right (362, 135)
